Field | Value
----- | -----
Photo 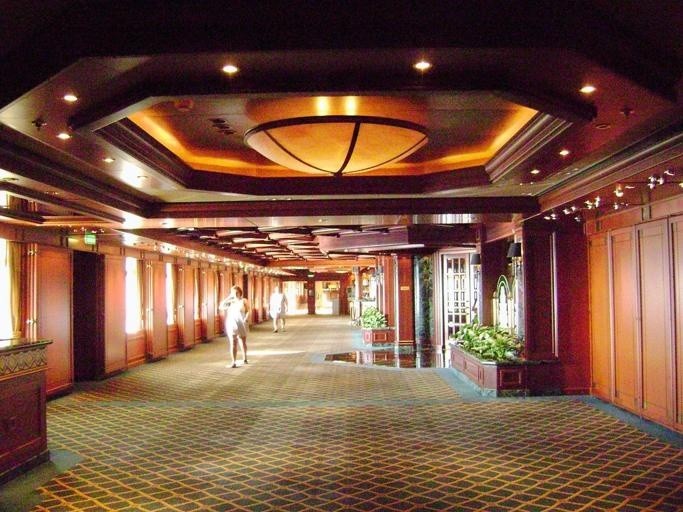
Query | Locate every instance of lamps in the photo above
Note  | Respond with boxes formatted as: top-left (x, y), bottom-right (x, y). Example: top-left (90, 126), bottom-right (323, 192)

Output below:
top-left (242, 93), bottom-right (432, 185)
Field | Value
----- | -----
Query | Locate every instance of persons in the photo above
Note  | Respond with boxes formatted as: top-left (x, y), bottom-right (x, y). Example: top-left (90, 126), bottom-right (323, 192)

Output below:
top-left (268, 285), bottom-right (289, 333)
top-left (217, 283), bottom-right (252, 369)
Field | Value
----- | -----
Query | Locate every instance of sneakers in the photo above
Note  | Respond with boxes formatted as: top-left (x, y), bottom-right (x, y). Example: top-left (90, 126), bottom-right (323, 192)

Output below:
top-left (232, 363), bottom-right (237, 368)
top-left (243, 360), bottom-right (248, 364)
top-left (273, 328), bottom-right (279, 333)
